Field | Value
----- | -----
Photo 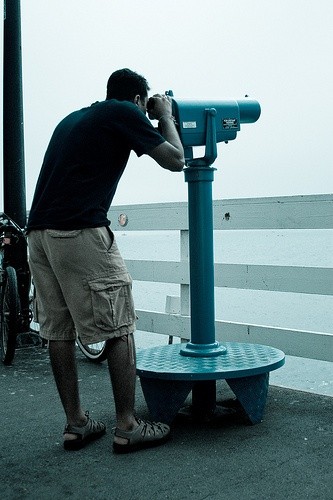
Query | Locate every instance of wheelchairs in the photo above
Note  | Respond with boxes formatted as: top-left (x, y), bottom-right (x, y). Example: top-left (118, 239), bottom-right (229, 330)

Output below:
top-left (0, 211), bottom-right (115, 365)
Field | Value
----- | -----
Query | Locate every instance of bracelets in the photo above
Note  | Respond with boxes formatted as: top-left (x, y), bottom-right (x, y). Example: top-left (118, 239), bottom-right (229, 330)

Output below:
top-left (159, 115), bottom-right (178, 125)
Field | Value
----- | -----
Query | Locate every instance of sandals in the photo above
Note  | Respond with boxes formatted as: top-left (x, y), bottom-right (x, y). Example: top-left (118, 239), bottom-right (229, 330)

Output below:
top-left (111, 421), bottom-right (171, 454)
top-left (61, 410), bottom-right (107, 451)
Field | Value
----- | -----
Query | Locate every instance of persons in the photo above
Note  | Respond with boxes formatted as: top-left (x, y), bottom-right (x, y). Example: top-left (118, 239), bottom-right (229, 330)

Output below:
top-left (25, 68), bottom-right (185, 452)
